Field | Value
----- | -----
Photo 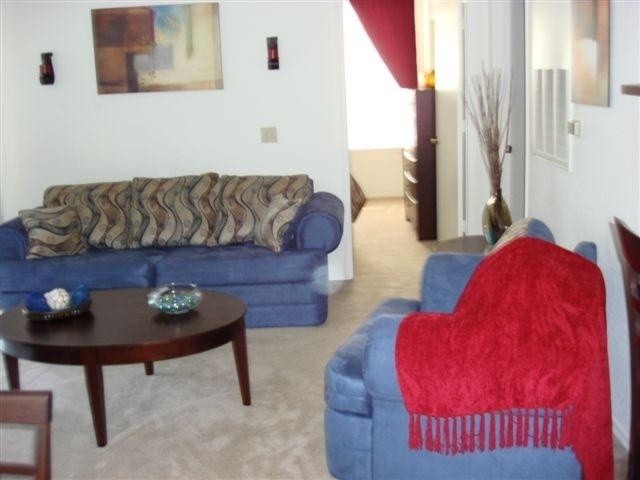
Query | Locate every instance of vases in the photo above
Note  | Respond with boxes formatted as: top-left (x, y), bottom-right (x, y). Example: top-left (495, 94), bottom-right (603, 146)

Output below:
top-left (481, 195), bottom-right (512, 247)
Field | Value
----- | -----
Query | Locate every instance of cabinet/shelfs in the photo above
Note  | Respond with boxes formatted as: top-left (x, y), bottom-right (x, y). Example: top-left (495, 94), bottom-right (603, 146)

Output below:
top-left (401, 92), bottom-right (436, 239)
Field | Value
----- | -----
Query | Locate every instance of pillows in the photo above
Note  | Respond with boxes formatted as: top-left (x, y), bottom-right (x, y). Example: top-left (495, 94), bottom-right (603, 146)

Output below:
top-left (17, 172), bottom-right (312, 261)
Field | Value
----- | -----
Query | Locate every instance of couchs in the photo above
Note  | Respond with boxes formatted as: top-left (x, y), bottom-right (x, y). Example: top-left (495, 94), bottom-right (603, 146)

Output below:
top-left (0, 192), bottom-right (345, 328)
top-left (323, 217), bottom-right (607, 479)
